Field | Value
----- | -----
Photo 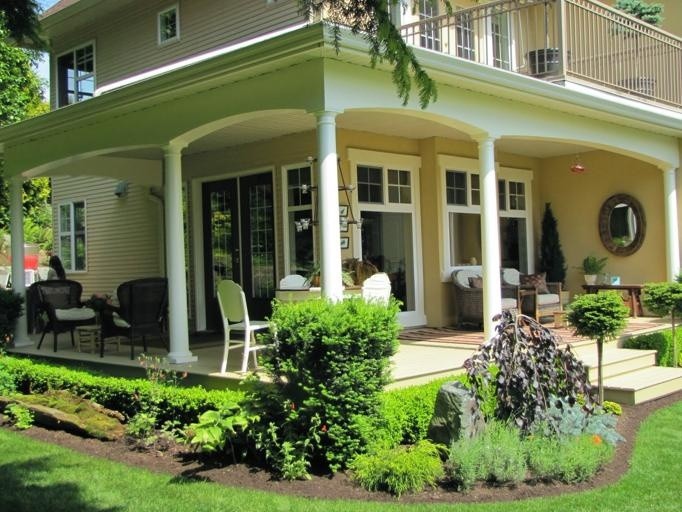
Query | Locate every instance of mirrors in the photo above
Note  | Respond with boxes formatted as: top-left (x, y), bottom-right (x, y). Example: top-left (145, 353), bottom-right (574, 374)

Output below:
top-left (598, 193), bottom-right (648, 256)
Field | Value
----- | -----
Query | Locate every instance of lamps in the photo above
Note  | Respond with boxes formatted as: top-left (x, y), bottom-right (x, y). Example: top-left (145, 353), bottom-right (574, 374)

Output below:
top-left (293, 154), bottom-right (319, 232)
top-left (337, 155), bottom-right (365, 229)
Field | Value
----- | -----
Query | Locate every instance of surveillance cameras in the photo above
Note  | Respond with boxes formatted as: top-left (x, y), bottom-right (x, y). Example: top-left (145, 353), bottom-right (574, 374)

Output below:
top-left (114, 181), bottom-right (125, 196)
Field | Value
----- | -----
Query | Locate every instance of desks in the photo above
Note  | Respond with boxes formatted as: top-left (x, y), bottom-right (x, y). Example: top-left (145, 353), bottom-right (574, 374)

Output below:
top-left (581, 283), bottom-right (646, 319)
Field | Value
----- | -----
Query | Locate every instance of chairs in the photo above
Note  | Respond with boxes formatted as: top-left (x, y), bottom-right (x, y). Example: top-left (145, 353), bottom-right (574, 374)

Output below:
top-left (30, 277), bottom-right (169, 361)
top-left (216, 272), bottom-right (392, 373)
top-left (450, 269), bottom-right (563, 330)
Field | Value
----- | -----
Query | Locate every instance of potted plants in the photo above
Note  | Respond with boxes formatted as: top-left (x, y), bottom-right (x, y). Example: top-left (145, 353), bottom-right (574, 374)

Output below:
top-left (572, 254), bottom-right (609, 285)
top-left (539, 201), bottom-right (570, 306)
top-left (523, 1), bottom-right (667, 99)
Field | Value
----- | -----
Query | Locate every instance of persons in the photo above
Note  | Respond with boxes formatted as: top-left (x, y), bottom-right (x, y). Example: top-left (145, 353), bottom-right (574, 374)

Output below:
top-left (46, 255), bottom-right (65, 282)
